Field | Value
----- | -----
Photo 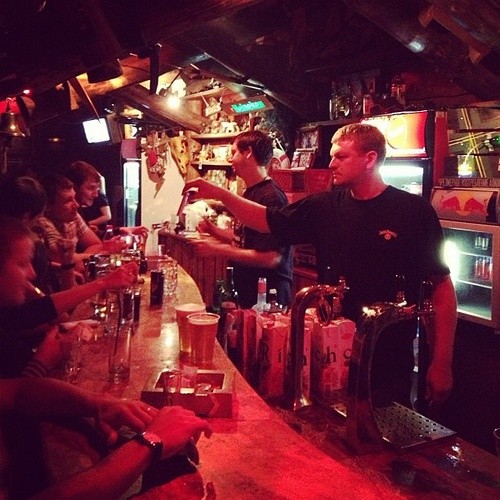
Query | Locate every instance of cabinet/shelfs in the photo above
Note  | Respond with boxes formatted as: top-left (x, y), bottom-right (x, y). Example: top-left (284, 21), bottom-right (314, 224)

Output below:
top-left (182, 83), bottom-right (284, 209)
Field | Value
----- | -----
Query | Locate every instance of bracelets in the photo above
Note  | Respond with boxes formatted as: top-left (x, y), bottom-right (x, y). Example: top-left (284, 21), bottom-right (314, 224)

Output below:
top-left (113, 226), bottom-right (121, 234)
top-left (60, 263), bottom-right (75, 269)
top-left (22, 359), bottom-right (48, 379)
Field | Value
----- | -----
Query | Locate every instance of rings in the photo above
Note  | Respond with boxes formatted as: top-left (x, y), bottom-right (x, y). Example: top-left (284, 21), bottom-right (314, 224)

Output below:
top-left (128, 272), bottom-right (133, 275)
top-left (146, 407), bottom-right (150, 412)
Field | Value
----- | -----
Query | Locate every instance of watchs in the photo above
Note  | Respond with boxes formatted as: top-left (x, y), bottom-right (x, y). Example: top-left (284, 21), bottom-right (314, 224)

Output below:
top-left (132, 431), bottom-right (163, 472)
top-left (32, 287), bottom-right (41, 297)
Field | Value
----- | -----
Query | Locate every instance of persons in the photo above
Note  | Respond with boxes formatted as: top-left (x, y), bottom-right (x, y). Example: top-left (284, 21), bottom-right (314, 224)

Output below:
top-left (182, 123), bottom-right (458, 411)
top-left (0, 161), bottom-right (148, 288)
top-left (0, 216), bottom-right (139, 378)
top-left (188, 131), bottom-right (292, 309)
top-left (0, 377), bottom-right (212, 500)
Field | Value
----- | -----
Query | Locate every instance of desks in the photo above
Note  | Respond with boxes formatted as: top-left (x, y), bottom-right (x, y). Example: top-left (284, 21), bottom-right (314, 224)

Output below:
top-left (158, 224), bottom-right (318, 307)
top-left (56, 256), bottom-right (404, 500)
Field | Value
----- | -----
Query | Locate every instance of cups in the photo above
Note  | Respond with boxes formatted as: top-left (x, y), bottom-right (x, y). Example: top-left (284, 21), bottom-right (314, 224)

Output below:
top-left (175, 302), bottom-right (221, 364)
top-left (61, 234), bottom-right (147, 385)
top-left (150, 259), bottom-right (179, 304)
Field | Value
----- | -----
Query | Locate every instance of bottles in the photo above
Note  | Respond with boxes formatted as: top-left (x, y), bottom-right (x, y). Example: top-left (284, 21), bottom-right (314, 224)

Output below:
top-left (219, 266), bottom-right (356, 403)
top-left (329, 77), bottom-right (409, 119)
top-left (104, 224), bottom-right (115, 240)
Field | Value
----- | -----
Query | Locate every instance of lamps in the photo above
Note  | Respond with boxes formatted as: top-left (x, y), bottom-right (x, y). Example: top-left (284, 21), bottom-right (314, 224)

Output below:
top-left (0, 60), bottom-right (204, 149)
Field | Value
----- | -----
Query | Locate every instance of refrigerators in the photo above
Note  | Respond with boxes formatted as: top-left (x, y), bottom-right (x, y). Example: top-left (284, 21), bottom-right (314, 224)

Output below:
top-left (360, 107), bottom-right (500, 449)
